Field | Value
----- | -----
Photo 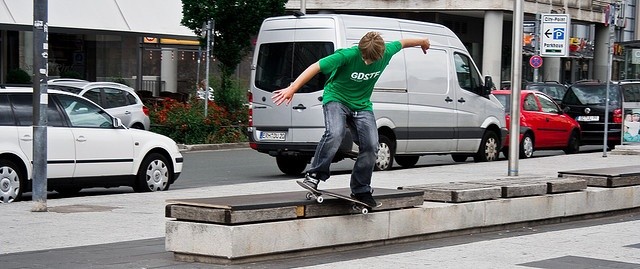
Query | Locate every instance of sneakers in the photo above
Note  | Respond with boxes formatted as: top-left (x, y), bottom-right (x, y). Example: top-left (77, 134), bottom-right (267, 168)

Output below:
top-left (350, 191), bottom-right (376, 207)
top-left (303, 173), bottom-right (319, 189)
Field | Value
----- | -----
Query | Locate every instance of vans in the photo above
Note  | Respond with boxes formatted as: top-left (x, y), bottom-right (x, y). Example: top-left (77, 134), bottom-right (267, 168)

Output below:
top-left (247, 13), bottom-right (507, 176)
top-left (561, 79), bottom-right (640, 149)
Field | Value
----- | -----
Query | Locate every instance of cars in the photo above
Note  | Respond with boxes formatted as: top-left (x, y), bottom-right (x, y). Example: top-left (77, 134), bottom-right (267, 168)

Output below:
top-left (451, 89), bottom-right (582, 162)
top-left (0, 84), bottom-right (183, 204)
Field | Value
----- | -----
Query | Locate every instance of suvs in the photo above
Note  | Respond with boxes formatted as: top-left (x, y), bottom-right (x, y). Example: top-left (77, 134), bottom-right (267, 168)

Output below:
top-left (501, 81), bottom-right (572, 105)
top-left (47, 78), bottom-right (151, 131)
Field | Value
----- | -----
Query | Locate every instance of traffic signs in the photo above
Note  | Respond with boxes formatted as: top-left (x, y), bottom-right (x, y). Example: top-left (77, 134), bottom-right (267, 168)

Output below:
top-left (540, 14), bottom-right (569, 57)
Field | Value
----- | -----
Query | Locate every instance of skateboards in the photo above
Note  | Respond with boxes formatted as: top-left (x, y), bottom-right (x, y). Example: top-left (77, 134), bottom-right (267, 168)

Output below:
top-left (296, 180), bottom-right (382, 215)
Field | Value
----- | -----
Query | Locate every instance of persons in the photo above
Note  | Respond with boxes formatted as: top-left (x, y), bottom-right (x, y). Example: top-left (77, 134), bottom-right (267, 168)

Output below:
top-left (624, 113), bottom-right (640, 142)
top-left (197, 80), bottom-right (215, 102)
top-left (624, 113), bottom-right (633, 134)
top-left (271, 32), bottom-right (430, 210)
